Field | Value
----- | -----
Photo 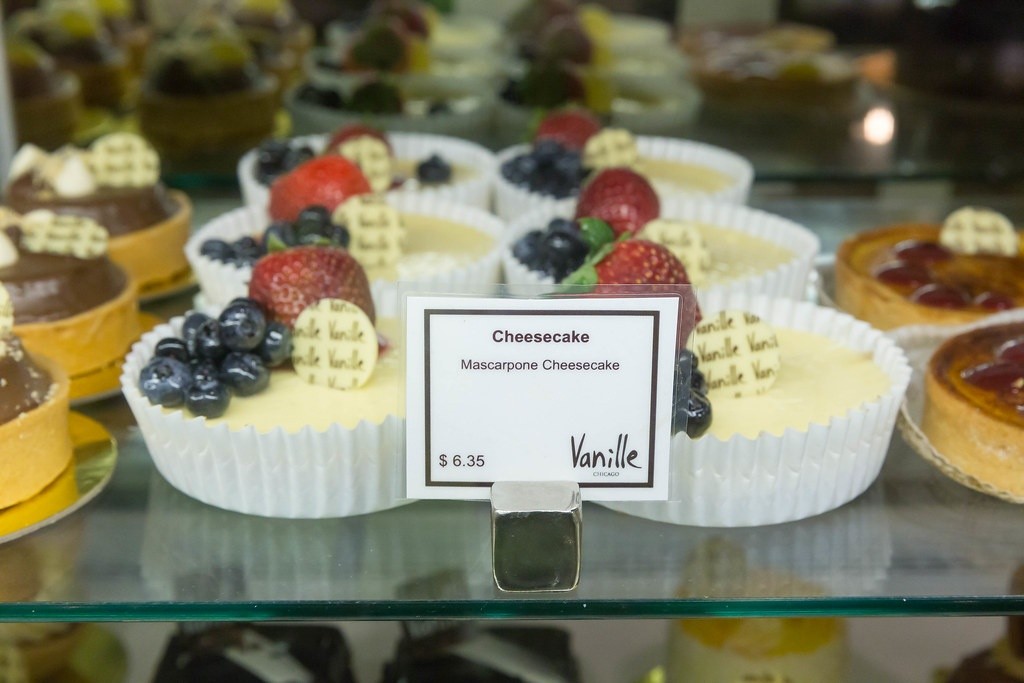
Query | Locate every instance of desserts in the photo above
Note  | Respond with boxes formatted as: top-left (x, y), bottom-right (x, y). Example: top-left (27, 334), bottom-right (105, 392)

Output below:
top-left (0, 0), bottom-right (881, 175)
top-left (0, 126), bottom-right (1024, 683)
